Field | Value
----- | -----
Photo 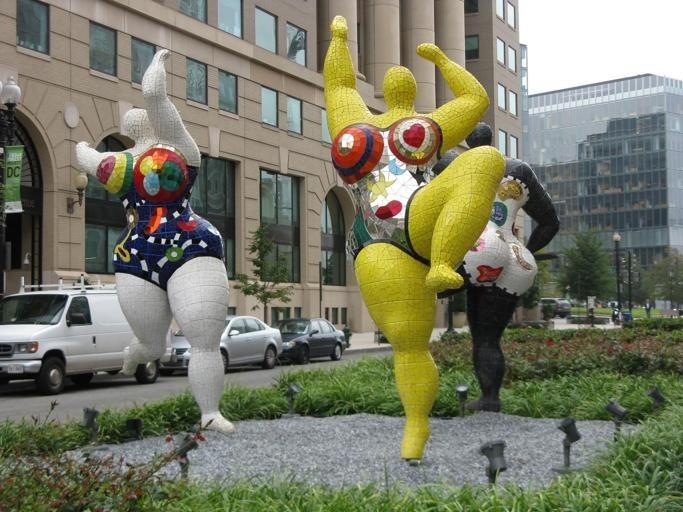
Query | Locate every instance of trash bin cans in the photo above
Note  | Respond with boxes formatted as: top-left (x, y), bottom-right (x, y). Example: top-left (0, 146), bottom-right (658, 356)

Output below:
top-left (622, 313), bottom-right (632, 321)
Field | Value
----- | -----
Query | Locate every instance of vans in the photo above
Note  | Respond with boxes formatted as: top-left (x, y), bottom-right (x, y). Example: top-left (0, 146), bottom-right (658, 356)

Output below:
top-left (0, 289), bottom-right (160, 395)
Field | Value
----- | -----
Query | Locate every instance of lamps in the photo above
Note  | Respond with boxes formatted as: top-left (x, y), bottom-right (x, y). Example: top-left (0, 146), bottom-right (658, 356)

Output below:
top-left (81, 407), bottom-right (99, 430)
top-left (605, 400), bottom-right (627, 417)
top-left (283, 383), bottom-right (299, 400)
top-left (67, 169), bottom-right (88, 213)
top-left (455, 385), bottom-right (469, 401)
top-left (648, 386), bottom-right (665, 405)
top-left (481, 441), bottom-right (506, 475)
top-left (560, 417), bottom-right (581, 444)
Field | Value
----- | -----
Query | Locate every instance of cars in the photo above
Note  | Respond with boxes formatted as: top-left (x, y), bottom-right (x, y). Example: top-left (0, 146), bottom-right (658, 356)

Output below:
top-left (270, 317), bottom-right (347, 365)
top-left (538, 297), bottom-right (571, 318)
top-left (158, 315), bottom-right (283, 377)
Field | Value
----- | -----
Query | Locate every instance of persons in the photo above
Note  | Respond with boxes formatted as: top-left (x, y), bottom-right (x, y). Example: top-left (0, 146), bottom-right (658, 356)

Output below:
top-left (643, 298), bottom-right (652, 319)
top-left (610, 307), bottom-right (621, 327)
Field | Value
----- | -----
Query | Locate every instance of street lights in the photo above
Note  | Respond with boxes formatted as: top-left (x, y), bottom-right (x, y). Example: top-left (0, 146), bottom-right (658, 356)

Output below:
top-left (612, 234), bottom-right (621, 311)
top-left (1, 74), bottom-right (23, 286)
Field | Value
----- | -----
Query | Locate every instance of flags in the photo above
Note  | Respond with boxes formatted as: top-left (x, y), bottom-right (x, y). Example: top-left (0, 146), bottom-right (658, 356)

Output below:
top-left (1, 144), bottom-right (28, 217)
